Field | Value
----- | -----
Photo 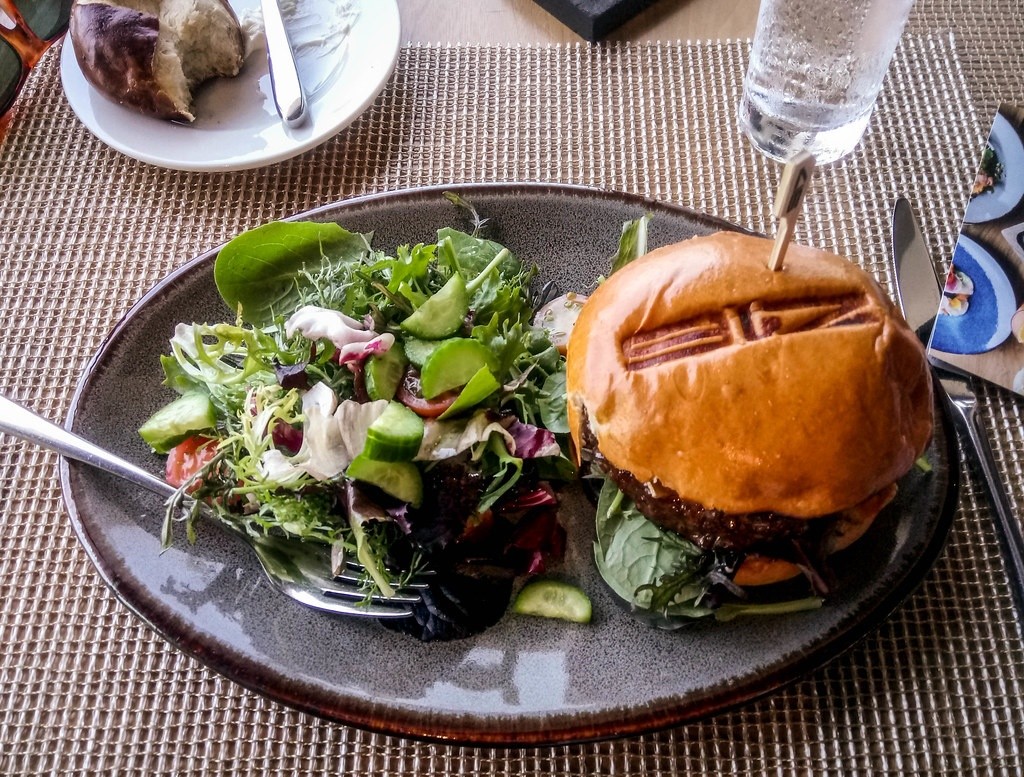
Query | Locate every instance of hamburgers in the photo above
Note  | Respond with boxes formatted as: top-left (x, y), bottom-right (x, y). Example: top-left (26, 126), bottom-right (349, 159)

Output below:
top-left (568, 231), bottom-right (935, 591)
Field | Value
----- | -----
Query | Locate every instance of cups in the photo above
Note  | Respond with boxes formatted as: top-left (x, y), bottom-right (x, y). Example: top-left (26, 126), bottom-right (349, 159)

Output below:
top-left (739, 1), bottom-right (914, 167)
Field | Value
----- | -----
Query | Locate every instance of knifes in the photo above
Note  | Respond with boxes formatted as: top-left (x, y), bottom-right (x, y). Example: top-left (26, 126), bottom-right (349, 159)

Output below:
top-left (890, 195), bottom-right (1024, 625)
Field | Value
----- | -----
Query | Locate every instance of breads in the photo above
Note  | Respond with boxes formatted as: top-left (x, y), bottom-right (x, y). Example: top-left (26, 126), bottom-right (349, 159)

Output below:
top-left (67, 0), bottom-right (251, 124)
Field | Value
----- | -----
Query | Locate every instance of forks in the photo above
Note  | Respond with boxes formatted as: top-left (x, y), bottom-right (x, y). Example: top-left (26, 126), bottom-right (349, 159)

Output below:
top-left (0, 395), bottom-right (436, 621)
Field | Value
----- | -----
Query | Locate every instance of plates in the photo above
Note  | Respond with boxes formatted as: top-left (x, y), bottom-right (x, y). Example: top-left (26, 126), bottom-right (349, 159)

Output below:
top-left (58, 0), bottom-right (402, 175)
top-left (57, 177), bottom-right (963, 755)
